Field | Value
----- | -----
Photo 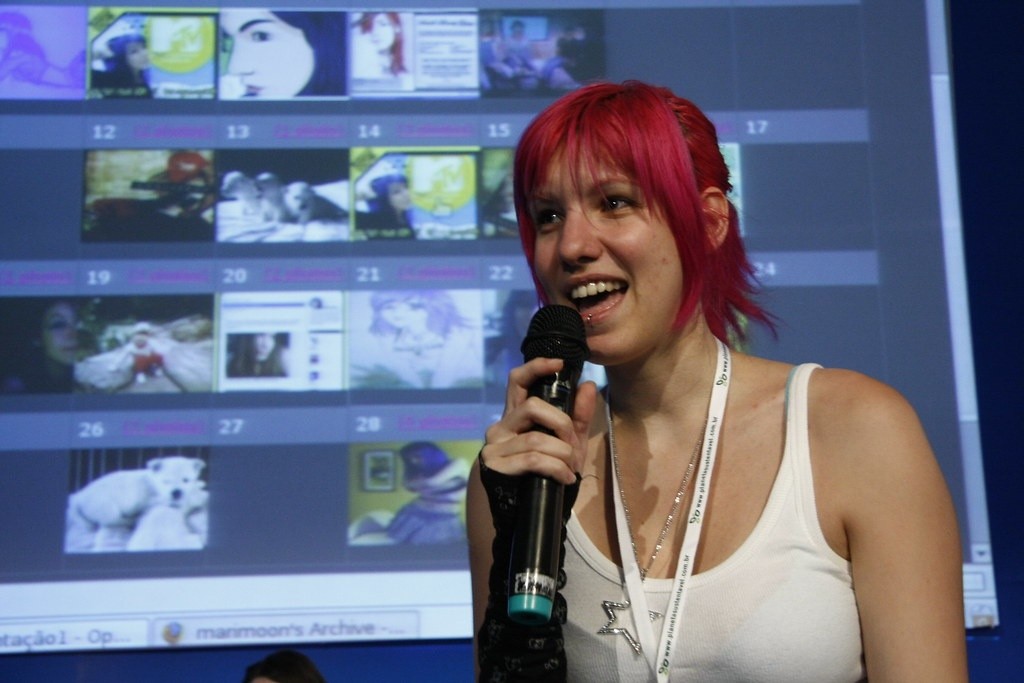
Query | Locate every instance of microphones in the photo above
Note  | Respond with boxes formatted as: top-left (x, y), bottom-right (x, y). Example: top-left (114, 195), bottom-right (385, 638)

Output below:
top-left (507, 302), bottom-right (593, 626)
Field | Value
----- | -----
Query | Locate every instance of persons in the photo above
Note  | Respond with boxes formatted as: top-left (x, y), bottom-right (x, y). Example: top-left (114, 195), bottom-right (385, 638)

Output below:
top-left (465, 80), bottom-right (970, 683)
top-left (0, 0), bottom-right (602, 544)
top-left (240, 650), bottom-right (329, 683)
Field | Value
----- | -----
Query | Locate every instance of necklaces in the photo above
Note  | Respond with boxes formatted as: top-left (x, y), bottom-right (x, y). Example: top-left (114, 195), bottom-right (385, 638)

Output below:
top-left (597, 390), bottom-right (714, 655)
